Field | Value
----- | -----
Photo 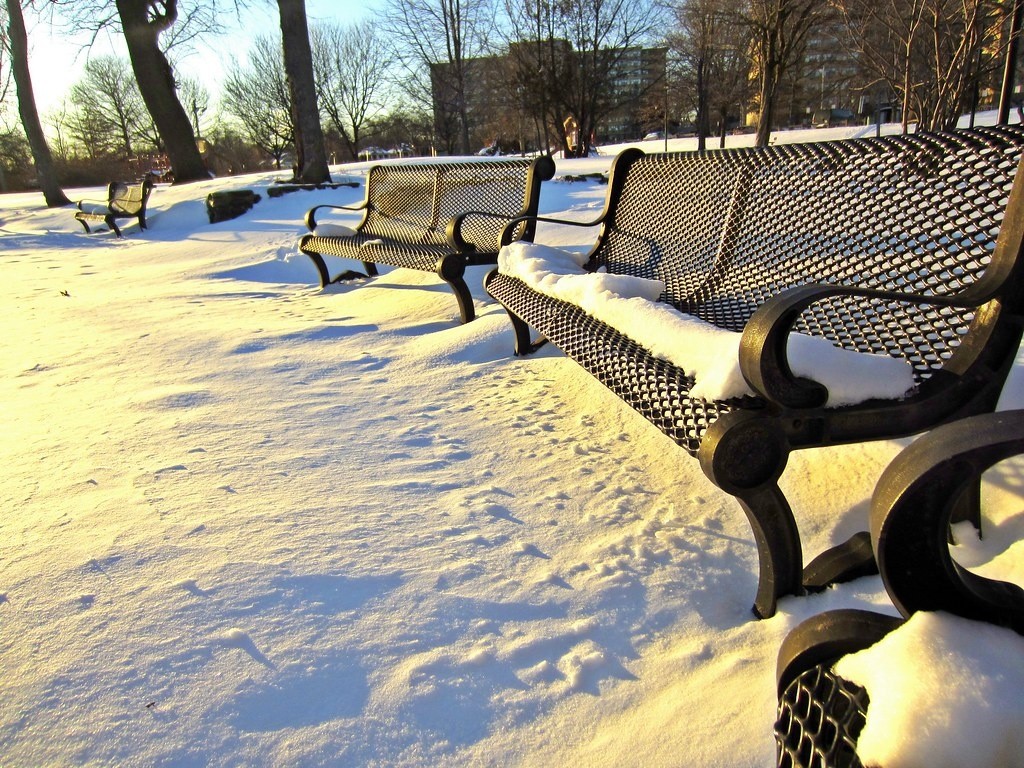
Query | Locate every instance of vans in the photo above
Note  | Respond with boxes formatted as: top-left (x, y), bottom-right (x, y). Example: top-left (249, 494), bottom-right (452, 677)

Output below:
top-left (811, 107), bottom-right (858, 128)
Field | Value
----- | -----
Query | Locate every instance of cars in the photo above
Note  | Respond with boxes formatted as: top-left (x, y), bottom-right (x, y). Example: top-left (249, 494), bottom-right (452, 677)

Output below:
top-left (643, 131), bottom-right (665, 141)
top-left (357, 145), bottom-right (388, 162)
top-left (733, 126), bottom-right (755, 135)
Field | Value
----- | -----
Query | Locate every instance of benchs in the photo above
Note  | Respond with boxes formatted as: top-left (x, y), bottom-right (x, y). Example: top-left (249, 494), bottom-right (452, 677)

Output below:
top-left (481, 123), bottom-right (1023, 621)
top-left (75, 180), bottom-right (157, 238)
top-left (774, 409), bottom-right (1024, 768)
top-left (299, 156), bottom-right (556, 325)
